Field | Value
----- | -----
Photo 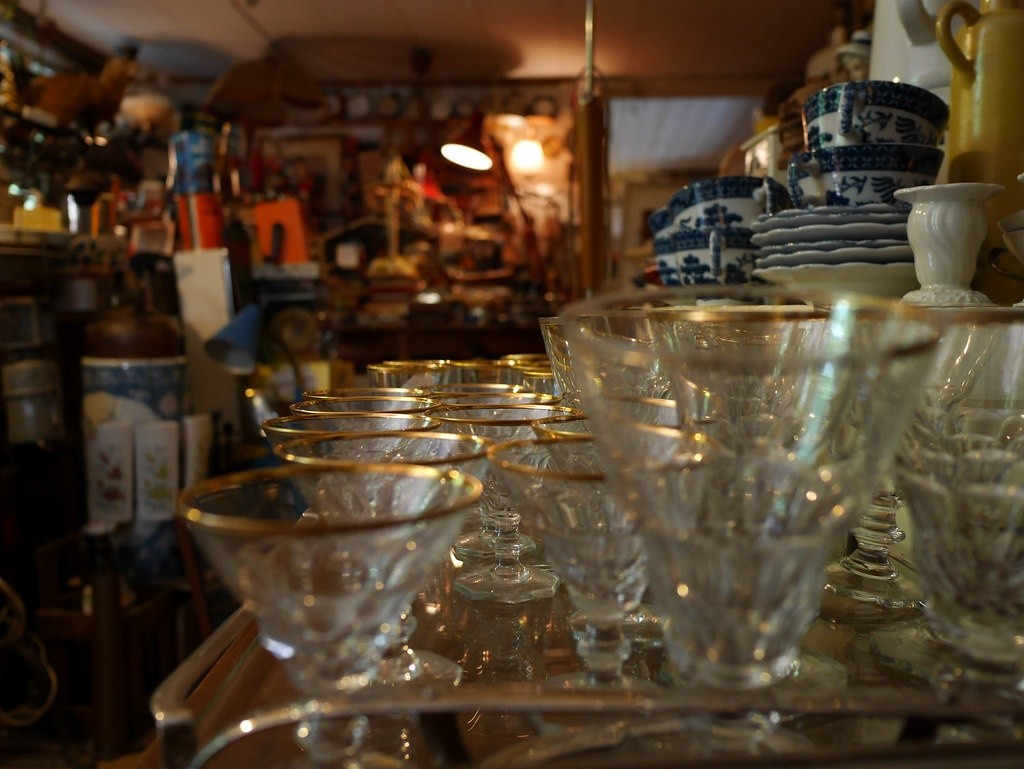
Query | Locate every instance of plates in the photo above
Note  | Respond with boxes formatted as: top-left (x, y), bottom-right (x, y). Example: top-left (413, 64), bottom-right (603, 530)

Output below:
top-left (752, 210), bottom-right (922, 312)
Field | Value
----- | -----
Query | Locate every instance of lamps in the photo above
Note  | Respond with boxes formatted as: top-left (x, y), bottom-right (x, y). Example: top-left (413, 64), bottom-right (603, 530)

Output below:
top-left (438, 103), bottom-right (549, 296)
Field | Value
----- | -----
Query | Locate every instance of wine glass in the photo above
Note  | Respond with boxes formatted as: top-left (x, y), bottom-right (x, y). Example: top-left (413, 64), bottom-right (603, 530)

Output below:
top-left (180, 288), bottom-right (1024, 769)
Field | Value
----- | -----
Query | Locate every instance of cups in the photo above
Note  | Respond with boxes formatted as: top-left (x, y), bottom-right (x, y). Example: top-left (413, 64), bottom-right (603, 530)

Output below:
top-left (786, 145), bottom-right (945, 213)
top-left (801, 80), bottom-right (951, 152)
top-left (648, 206), bottom-right (672, 241)
top-left (651, 238), bottom-right (681, 286)
top-left (668, 176), bottom-right (792, 232)
top-left (672, 232), bottom-right (755, 286)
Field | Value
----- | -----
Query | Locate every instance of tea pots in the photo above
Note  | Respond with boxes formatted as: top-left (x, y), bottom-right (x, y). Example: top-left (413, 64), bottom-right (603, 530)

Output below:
top-left (936, 0), bottom-right (1024, 306)
